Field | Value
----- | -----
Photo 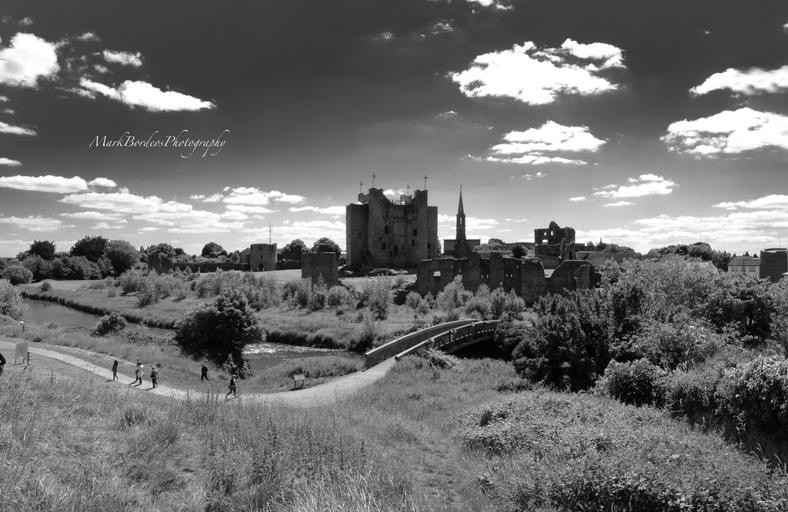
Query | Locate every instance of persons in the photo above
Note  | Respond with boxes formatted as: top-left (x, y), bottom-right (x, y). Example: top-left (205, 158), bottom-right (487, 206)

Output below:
top-left (226, 374), bottom-right (238, 398)
top-left (135, 357), bottom-right (141, 382)
top-left (26, 351), bottom-right (32, 365)
top-left (112, 359), bottom-right (120, 381)
top-left (137, 362), bottom-right (144, 384)
top-left (0, 353), bottom-right (6, 376)
top-left (201, 363), bottom-right (211, 381)
top-left (150, 365), bottom-right (158, 388)
